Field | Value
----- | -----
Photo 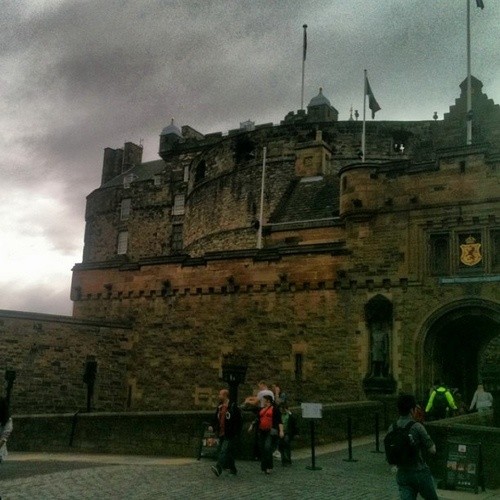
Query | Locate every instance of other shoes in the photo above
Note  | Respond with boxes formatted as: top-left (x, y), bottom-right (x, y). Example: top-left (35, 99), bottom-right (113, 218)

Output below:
top-left (211, 466), bottom-right (222, 477)
top-left (266, 469), bottom-right (272, 474)
top-left (226, 472), bottom-right (237, 476)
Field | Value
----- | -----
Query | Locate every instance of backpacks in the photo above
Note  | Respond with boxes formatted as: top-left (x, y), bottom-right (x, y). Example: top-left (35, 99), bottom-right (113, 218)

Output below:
top-left (432, 389), bottom-right (448, 410)
top-left (383, 420), bottom-right (418, 466)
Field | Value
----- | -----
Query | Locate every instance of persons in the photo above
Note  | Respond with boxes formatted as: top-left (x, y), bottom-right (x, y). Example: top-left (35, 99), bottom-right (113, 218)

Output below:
top-left (425, 379), bottom-right (494, 420)
top-left (244, 379), bottom-right (296, 473)
top-left (0, 398), bottom-right (12, 500)
top-left (207, 389), bottom-right (238, 477)
top-left (387, 394), bottom-right (441, 500)
